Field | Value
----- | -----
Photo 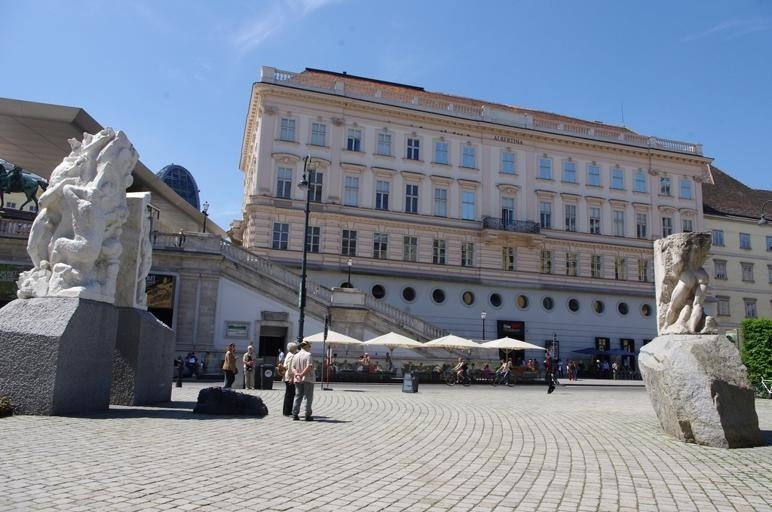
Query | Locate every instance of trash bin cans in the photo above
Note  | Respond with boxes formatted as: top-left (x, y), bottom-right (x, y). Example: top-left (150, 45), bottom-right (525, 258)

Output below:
top-left (260, 364), bottom-right (275, 390)
top-left (402, 370), bottom-right (418, 393)
top-left (255, 358), bottom-right (265, 389)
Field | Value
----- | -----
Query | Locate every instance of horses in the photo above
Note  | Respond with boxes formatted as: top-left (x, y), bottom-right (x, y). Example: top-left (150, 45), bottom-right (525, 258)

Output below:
top-left (0, 164), bottom-right (49, 213)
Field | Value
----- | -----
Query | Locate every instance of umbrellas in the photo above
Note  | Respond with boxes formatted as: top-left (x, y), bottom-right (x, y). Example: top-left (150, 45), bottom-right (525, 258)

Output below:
top-left (424, 332), bottom-right (486, 357)
top-left (480, 334), bottom-right (546, 362)
top-left (294, 329), bottom-right (361, 357)
top-left (360, 331), bottom-right (426, 357)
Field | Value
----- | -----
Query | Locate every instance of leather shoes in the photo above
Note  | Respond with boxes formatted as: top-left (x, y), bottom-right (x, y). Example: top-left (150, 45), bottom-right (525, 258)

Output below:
top-left (292, 415), bottom-right (300, 420)
top-left (305, 415), bottom-right (313, 421)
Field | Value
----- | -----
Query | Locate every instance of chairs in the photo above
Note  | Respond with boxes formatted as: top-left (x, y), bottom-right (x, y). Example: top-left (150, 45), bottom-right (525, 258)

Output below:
top-left (471, 370), bottom-right (498, 384)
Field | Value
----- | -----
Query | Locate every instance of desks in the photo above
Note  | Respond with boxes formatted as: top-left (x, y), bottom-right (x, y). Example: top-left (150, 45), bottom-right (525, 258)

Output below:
top-left (524, 372), bottom-right (539, 384)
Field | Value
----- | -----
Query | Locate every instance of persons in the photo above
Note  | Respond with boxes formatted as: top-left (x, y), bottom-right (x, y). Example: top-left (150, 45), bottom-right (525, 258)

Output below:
top-left (360, 353), bottom-right (391, 371)
top-left (496, 358), bottom-right (513, 380)
top-left (544, 352), bottom-right (553, 387)
top-left (484, 365), bottom-right (492, 377)
top-left (406, 362), bottom-right (448, 373)
top-left (222, 343), bottom-right (240, 390)
top-left (4, 164), bottom-right (23, 195)
top-left (278, 342), bottom-right (314, 422)
top-left (243, 345), bottom-right (256, 390)
top-left (599, 360), bottom-right (620, 380)
top-left (176, 351), bottom-right (199, 387)
top-left (521, 359), bottom-right (539, 368)
top-left (331, 354), bottom-right (337, 362)
top-left (567, 361), bottom-right (578, 381)
top-left (452, 358), bottom-right (466, 383)
